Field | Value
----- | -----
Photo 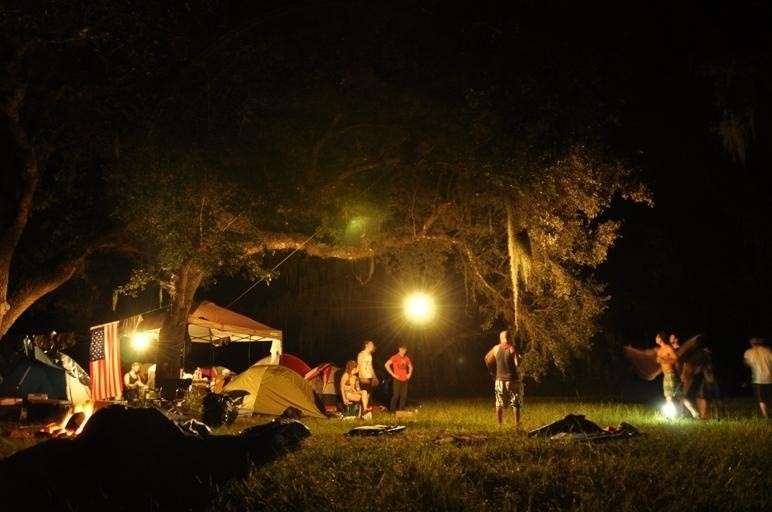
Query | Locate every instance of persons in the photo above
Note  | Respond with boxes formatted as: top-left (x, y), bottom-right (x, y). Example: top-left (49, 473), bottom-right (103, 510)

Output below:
top-left (624, 331), bottom-right (771, 420)
top-left (120, 360), bottom-right (237, 401)
top-left (354, 338), bottom-right (380, 408)
top-left (483, 330), bottom-right (526, 429)
top-left (517, 413), bottom-right (629, 439)
top-left (339, 360), bottom-right (373, 413)
top-left (383, 344), bottom-right (415, 415)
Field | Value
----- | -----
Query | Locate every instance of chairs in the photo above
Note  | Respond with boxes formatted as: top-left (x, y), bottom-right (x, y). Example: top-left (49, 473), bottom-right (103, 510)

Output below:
top-left (158, 378), bottom-right (192, 414)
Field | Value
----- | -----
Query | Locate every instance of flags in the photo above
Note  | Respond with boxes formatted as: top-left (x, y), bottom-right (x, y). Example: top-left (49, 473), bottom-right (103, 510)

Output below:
top-left (84, 321), bottom-right (125, 402)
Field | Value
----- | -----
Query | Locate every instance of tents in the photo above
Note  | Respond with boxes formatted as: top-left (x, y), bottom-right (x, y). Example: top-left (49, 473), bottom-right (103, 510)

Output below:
top-left (216, 363), bottom-right (329, 420)
top-left (0, 335), bottom-right (91, 401)
top-left (246, 352), bottom-right (312, 379)
top-left (303, 361), bottom-right (382, 413)
